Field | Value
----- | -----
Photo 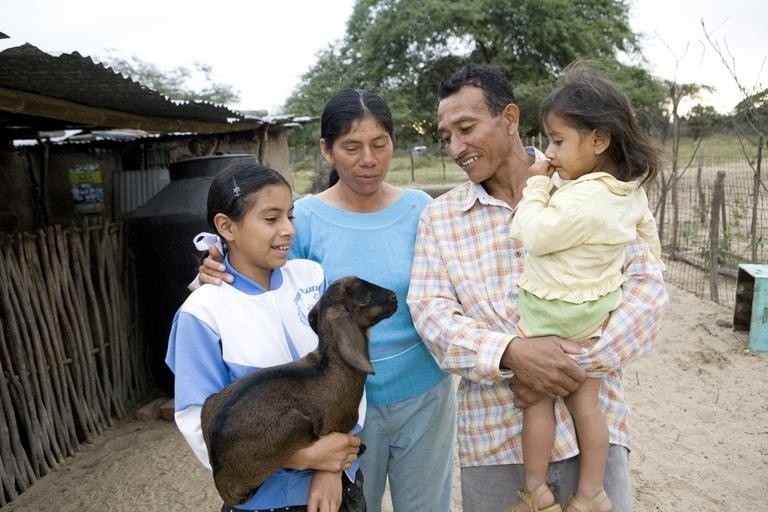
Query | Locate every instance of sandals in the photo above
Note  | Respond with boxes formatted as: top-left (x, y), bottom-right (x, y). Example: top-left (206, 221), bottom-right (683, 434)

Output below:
top-left (563, 488), bottom-right (617, 512)
top-left (506, 482), bottom-right (563, 512)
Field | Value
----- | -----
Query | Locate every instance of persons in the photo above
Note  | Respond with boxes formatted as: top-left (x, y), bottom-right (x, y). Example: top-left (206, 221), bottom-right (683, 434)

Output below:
top-left (408, 61), bottom-right (667, 510)
top-left (506, 75), bottom-right (666, 510)
top-left (195, 87), bottom-right (459, 512)
top-left (166, 157), bottom-right (370, 510)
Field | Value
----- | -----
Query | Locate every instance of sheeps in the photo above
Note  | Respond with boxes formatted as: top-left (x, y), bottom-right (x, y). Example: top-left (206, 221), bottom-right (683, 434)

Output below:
top-left (200, 276), bottom-right (398, 506)
